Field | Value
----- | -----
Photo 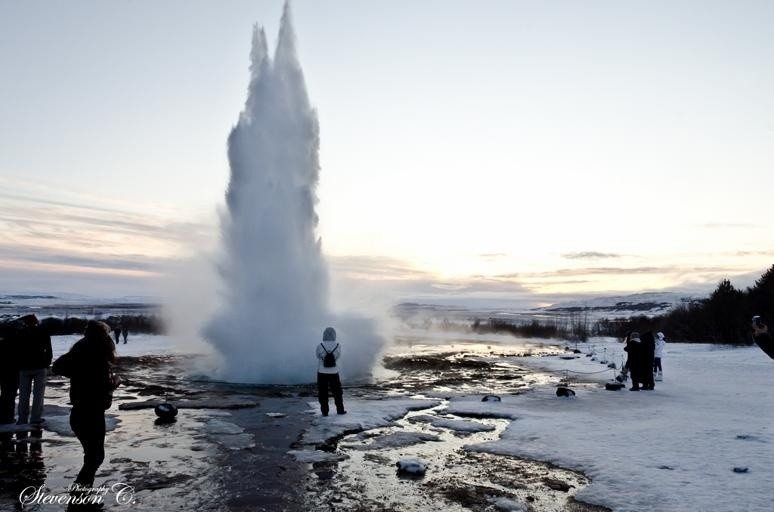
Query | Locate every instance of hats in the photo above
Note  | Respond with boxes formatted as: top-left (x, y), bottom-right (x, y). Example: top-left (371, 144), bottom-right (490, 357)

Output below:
top-left (629, 332), bottom-right (641, 343)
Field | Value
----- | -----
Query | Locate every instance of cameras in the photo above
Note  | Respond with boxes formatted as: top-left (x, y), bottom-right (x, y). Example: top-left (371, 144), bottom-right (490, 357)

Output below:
top-left (753, 316), bottom-right (761, 329)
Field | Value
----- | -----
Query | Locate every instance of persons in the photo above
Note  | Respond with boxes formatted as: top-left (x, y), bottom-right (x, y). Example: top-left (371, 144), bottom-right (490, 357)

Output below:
top-left (0, 315), bottom-right (130, 496)
top-left (616, 329), bottom-right (666, 391)
top-left (316, 326), bottom-right (347, 417)
top-left (751, 323), bottom-right (774, 359)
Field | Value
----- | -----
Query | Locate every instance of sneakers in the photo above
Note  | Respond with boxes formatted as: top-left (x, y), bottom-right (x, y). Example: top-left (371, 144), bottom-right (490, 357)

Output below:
top-left (629, 384), bottom-right (654, 391)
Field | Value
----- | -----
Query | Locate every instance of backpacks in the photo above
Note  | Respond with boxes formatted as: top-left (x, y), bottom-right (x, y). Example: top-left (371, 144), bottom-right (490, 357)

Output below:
top-left (320, 342), bottom-right (339, 367)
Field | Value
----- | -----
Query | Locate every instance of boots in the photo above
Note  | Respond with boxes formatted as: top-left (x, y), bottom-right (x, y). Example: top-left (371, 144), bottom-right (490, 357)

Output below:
top-left (653, 371), bottom-right (663, 381)
